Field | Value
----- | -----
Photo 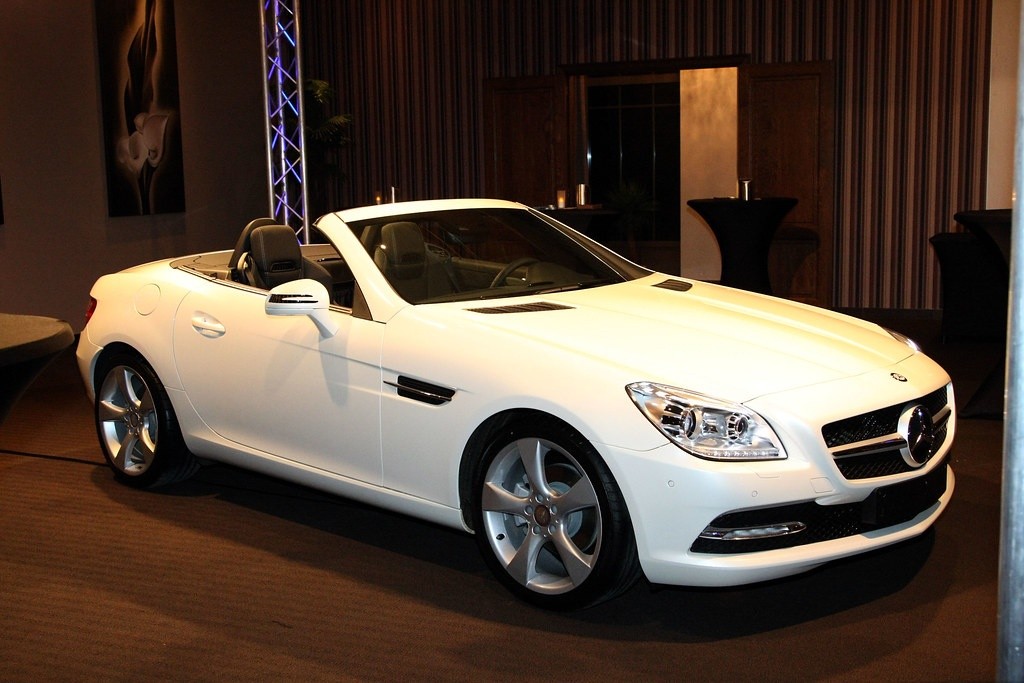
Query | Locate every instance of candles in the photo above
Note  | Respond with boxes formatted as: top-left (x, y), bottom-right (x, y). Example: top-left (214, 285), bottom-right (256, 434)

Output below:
top-left (557, 190), bottom-right (566, 209)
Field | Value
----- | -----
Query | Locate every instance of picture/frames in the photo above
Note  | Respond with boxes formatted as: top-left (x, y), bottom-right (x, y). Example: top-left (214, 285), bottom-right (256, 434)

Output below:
top-left (95, 0), bottom-right (186, 217)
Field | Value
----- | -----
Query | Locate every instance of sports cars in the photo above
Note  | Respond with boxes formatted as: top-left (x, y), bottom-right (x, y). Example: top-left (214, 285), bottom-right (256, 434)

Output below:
top-left (70, 197), bottom-right (963, 621)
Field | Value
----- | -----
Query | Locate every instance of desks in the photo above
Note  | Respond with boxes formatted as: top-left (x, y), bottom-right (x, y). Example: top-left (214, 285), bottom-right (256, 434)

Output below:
top-left (687, 195), bottom-right (799, 296)
top-left (543, 208), bottom-right (621, 240)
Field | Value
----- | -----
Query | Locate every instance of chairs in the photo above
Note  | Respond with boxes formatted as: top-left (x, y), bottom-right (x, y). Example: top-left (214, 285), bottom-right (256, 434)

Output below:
top-left (359, 220), bottom-right (455, 303)
top-left (227, 217), bottom-right (334, 305)
top-left (929, 207), bottom-right (1013, 419)
